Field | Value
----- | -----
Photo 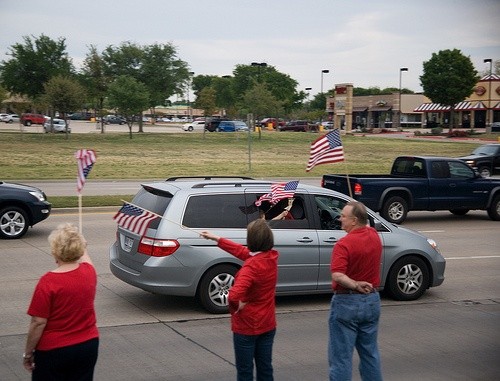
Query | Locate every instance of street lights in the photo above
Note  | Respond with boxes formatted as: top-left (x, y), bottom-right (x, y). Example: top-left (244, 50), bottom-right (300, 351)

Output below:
top-left (320, 69), bottom-right (330, 121)
top-left (253, 62), bottom-right (268, 133)
top-left (484, 59), bottom-right (492, 126)
top-left (398, 68), bottom-right (409, 127)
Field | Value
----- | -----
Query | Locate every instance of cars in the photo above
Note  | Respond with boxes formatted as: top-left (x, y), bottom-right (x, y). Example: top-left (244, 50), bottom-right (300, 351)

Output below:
top-left (3, 115), bottom-right (20, 124)
top-left (0, 180), bottom-right (53, 239)
top-left (181, 121), bottom-right (205, 132)
top-left (142, 117), bottom-right (188, 123)
top-left (255, 118), bottom-right (284, 129)
top-left (0, 114), bottom-right (9, 122)
top-left (204, 118), bottom-right (249, 133)
top-left (321, 122), bottom-right (334, 131)
top-left (449, 143), bottom-right (500, 178)
top-left (43, 118), bottom-right (71, 134)
top-left (102, 115), bottom-right (127, 125)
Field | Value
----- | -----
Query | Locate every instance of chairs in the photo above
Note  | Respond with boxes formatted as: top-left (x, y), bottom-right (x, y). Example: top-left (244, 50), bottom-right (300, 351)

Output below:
top-left (273, 197), bottom-right (306, 220)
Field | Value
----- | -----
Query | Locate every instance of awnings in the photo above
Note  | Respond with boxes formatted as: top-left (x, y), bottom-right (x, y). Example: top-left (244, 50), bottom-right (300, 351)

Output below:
top-left (413, 102), bottom-right (473, 112)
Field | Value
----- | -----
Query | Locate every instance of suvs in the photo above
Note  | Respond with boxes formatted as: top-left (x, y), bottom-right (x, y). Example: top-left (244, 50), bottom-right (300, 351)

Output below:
top-left (20, 113), bottom-right (49, 126)
top-left (110, 175), bottom-right (447, 315)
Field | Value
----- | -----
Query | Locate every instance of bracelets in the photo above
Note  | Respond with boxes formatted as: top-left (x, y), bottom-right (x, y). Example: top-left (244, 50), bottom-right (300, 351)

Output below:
top-left (23, 353), bottom-right (32, 359)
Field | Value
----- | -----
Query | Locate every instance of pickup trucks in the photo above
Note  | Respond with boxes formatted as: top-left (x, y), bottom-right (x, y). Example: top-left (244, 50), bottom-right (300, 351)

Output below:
top-left (279, 120), bottom-right (318, 132)
top-left (321, 156), bottom-right (500, 225)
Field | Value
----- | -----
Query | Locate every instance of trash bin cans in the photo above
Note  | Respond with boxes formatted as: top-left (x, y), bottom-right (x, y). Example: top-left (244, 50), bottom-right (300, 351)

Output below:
top-left (491, 122), bottom-right (500, 132)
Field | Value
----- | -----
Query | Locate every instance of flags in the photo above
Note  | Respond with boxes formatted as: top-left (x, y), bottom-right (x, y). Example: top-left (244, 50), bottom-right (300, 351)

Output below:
top-left (113, 203), bottom-right (159, 235)
top-left (74, 149), bottom-right (97, 192)
top-left (255, 180), bottom-right (300, 206)
top-left (306, 128), bottom-right (344, 172)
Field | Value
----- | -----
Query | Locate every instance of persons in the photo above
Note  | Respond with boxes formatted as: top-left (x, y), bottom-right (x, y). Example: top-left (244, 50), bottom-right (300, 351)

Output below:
top-left (200, 219), bottom-right (279, 381)
top-left (259, 209), bottom-right (288, 229)
top-left (328, 201), bottom-right (382, 381)
top-left (22, 223), bottom-right (100, 381)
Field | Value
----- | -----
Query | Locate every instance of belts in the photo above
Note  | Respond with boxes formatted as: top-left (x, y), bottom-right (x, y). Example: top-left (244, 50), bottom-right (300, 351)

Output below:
top-left (334, 288), bottom-right (362, 294)
